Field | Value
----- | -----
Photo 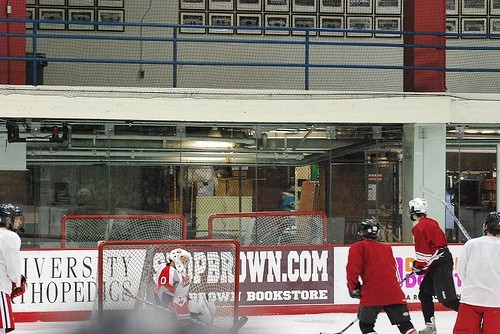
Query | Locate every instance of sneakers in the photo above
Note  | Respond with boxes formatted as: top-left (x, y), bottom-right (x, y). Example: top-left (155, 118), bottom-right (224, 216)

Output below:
top-left (419, 317), bottom-right (436, 334)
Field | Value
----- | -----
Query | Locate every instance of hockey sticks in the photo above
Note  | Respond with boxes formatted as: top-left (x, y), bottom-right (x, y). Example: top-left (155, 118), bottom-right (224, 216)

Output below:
top-left (122, 287), bottom-right (248, 334)
top-left (320, 271), bottom-right (415, 334)
top-left (422, 189), bottom-right (472, 241)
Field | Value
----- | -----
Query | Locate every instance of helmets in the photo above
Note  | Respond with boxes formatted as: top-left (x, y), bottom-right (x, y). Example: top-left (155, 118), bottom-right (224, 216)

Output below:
top-left (408, 198), bottom-right (428, 214)
top-left (357, 219), bottom-right (379, 239)
top-left (0, 203), bottom-right (25, 229)
top-left (169, 248), bottom-right (192, 274)
top-left (485, 211), bottom-right (500, 231)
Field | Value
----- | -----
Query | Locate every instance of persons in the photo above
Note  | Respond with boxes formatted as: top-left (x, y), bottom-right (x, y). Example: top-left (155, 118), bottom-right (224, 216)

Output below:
top-left (408, 197), bottom-right (500, 334)
top-left (0, 204), bottom-right (216, 334)
top-left (347, 219), bottom-right (419, 334)
top-left (52, 187), bottom-right (127, 242)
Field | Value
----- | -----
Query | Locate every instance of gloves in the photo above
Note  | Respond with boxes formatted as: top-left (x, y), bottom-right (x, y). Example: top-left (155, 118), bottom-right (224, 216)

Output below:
top-left (9, 276), bottom-right (27, 300)
top-left (413, 267), bottom-right (422, 275)
top-left (347, 281), bottom-right (360, 298)
